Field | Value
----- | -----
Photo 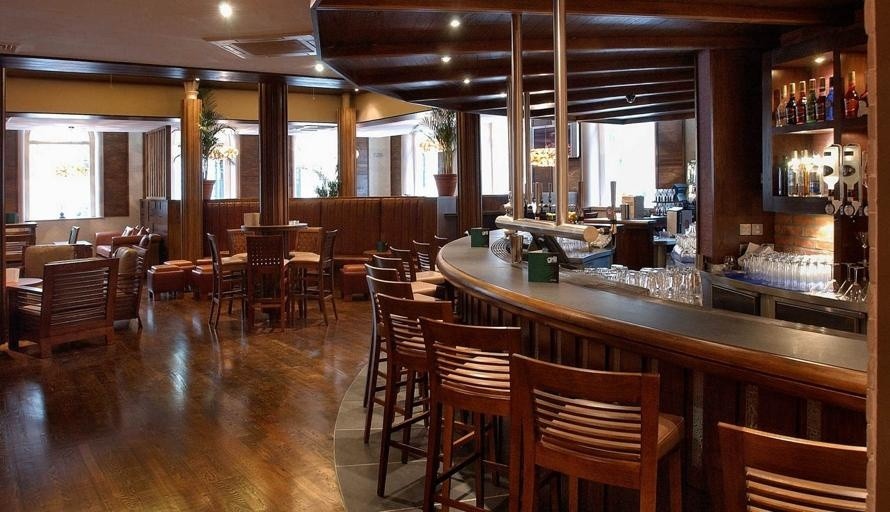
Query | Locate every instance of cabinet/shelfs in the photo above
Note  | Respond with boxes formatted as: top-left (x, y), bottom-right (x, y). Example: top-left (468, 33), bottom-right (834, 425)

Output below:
top-left (655, 121), bottom-right (696, 189)
top-left (761, 34), bottom-right (869, 212)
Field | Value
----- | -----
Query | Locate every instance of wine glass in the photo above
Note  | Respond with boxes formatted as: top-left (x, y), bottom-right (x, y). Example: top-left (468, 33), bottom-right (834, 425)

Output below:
top-left (723, 255), bottom-right (736, 275)
top-left (825, 260), bottom-right (869, 303)
top-left (581, 264), bottom-right (704, 306)
top-left (653, 188), bottom-right (675, 202)
top-left (673, 222), bottom-right (697, 259)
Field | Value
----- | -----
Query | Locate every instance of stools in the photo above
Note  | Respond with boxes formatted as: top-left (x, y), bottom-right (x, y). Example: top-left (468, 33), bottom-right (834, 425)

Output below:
top-left (146, 249), bottom-right (418, 302)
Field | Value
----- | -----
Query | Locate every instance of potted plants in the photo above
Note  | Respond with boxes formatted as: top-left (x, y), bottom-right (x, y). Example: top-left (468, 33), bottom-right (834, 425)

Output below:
top-left (171, 87), bottom-right (238, 199)
top-left (409, 107), bottom-right (457, 196)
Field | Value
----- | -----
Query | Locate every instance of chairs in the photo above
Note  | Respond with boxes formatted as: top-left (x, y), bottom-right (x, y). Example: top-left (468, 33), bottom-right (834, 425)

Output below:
top-left (207, 227), bottom-right (338, 332)
top-left (3, 222), bottom-right (152, 359)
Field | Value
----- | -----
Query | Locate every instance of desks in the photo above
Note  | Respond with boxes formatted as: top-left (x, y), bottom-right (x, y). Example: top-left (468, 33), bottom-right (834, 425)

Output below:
top-left (55, 240), bottom-right (93, 258)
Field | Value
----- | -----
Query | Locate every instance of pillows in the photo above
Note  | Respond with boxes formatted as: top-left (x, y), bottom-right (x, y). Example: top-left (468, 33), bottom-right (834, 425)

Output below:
top-left (121, 225), bottom-right (152, 237)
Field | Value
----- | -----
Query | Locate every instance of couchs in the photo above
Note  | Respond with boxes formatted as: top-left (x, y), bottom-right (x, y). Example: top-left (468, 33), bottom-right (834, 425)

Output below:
top-left (94, 230), bottom-right (160, 278)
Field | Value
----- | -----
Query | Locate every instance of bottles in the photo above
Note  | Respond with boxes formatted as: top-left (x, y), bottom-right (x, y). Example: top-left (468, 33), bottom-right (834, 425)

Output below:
top-left (842, 145), bottom-right (860, 200)
top-left (778, 150), bottom-right (821, 197)
top-left (745, 248), bottom-right (832, 291)
top-left (806, 79), bottom-right (816, 123)
top-left (844, 69), bottom-right (858, 120)
top-left (826, 77), bottom-right (834, 120)
top-left (786, 83), bottom-right (797, 124)
top-left (816, 77), bottom-right (826, 122)
top-left (822, 146), bottom-right (839, 199)
top-left (776, 84), bottom-right (788, 127)
top-left (858, 71), bottom-right (868, 119)
top-left (796, 80), bottom-right (807, 124)
top-left (654, 202), bottom-right (673, 215)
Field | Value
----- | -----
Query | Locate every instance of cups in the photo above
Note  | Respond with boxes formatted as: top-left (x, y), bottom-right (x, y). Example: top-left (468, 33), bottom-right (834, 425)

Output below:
top-left (555, 235), bottom-right (590, 257)
